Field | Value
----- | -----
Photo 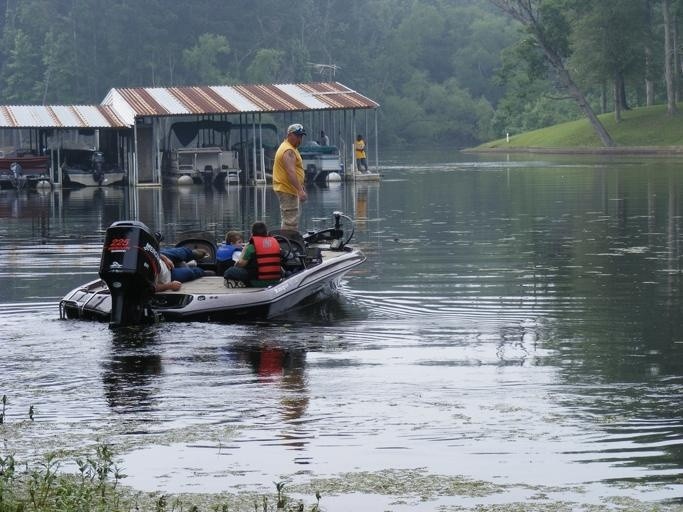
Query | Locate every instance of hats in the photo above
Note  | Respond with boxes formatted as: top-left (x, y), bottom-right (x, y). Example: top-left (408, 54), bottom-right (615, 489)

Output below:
top-left (287, 124), bottom-right (307, 136)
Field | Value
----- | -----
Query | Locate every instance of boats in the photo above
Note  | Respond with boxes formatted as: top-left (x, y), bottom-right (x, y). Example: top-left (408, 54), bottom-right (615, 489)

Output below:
top-left (59, 211), bottom-right (367, 323)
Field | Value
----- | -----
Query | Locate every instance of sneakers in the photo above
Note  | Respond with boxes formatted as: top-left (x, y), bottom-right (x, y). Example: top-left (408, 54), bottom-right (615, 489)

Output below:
top-left (357, 170), bottom-right (371, 174)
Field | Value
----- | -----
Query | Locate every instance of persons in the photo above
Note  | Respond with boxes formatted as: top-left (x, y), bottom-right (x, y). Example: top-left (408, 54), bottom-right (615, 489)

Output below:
top-left (246, 346), bottom-right (292, 385)
top-left (90, 149), bottom-right (106, 185)
top-left (271, 124), bottom-right (308, 232)
top-left (319, 131), bottom-right (329, 147)
top-left (277, 345), bottom-right (311, 450)
top-left (223, 221), bottom-right (284, 289)
top-left (353, 134), bottom-right (371, 175)
top-left (153, 230), bottom-right (216, 292)
top-left (354, 188), bottom-right (367, 231)
top-left (213, 230), bottom-right (249, 277)
